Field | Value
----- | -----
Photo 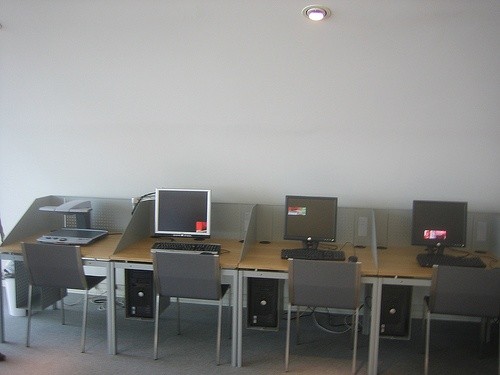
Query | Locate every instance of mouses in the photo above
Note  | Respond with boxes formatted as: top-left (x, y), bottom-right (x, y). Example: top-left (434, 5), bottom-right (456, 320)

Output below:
top-left (348, 256), bottom-right (358, 262)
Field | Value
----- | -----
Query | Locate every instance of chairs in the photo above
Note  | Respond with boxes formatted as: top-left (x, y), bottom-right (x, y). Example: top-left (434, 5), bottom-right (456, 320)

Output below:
top-left (151, 249), bottom-right (232, 366)
top-left (20, 242), bottom-right (107, 354)
top-left (421, 265), bottom-right (500, 375)
top-left (285, 258), bottom-right (366, 375)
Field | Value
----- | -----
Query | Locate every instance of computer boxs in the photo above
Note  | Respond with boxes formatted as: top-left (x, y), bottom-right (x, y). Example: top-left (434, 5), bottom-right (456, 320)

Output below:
top-left (248, 277), bottom-right (279, 327)
top-left (380, 284), bottom-right (411, 336)
top-left (125, 269), bottom-right (155, 318)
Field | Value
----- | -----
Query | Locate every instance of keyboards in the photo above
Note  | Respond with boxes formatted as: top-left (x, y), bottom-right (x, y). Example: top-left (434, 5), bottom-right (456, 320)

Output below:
top-left (151, 242), bottom-right (221, 255)
top-left (281, 248), bottom-right (345, 261)
top-left (417, 254), bottom-right (487, 268)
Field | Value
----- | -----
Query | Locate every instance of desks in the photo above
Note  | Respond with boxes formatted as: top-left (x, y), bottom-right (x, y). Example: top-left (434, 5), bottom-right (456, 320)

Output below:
top-left (0, 195), bottom-right (500, 375)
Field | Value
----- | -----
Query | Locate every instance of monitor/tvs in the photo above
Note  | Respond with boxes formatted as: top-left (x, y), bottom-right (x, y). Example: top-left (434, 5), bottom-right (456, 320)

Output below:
top-left (284, 196), bottom-right (337, 248)
top-left (411, 200), bottom-right (468, 253)
top-left (154, 189), bottom-right (211, 238)
